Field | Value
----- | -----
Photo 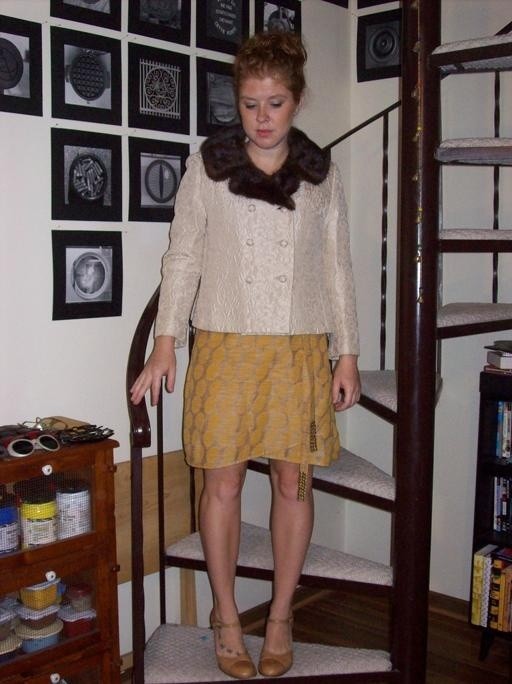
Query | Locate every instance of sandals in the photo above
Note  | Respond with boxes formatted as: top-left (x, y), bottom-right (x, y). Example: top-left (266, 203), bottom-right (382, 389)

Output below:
top-left (208, 608), bottom-right (256, 680)
top-left (258, 613), bottom-right (296, 678)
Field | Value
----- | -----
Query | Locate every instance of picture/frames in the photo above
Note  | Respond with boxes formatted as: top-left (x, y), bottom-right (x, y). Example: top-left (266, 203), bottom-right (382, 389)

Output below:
top-left (51, 230), bottom-right (123, 320)
top-left (0, 14), bottom-right (43, 117)
top-left (357, 8), bottom-right (401, 83)
top-left (128, 0), bottom-right (190, 47)
top-left (127, 42), bottom-right (190, 135)
top-left (196, 56), bottom-right (242, 137)
top-left (50, 0), bottom-right (121, 31)
top-left (128, 136), bottom-right (190, 222)
top-left (196, 0), bottom-right (249, 56)
top-left (50, 26), bottom-right (122, 126)
top-left (255, 0), bottom-right (301, 40)
top-left (51, 127), bottom-right (123, 222)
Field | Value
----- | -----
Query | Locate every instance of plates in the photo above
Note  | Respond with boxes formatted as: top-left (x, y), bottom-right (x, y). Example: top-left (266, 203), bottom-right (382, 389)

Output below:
top-left (209, 78), bottom-right (238, 123)
top-left (367, 27), bottom-right (399, 62)
top-left (63, 55), bottom-right (177, 301)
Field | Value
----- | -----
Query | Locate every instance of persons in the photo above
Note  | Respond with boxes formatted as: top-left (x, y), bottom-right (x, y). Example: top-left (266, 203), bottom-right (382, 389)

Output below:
top-left (130, 29), bottom-right (362, 679)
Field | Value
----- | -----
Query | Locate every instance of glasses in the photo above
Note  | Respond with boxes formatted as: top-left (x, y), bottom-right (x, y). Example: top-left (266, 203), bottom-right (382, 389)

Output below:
top-left (3, 434), bottom-right (61, 458)
top-left (61, 424), bottom-right (115, 443)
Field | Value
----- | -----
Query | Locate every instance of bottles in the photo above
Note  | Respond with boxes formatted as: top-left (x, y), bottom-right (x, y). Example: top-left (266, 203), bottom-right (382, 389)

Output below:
top-left (68, 586), bottom-right (93, 611)
top-left (0, 472), bottom-right (90, 555)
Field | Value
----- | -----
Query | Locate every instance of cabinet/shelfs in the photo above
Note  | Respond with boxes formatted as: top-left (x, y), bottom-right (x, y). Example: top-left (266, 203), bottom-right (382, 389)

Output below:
top-left (0, 428), bottom-right (120, 684)
top-left (468, 373), bottom-right (512, 661)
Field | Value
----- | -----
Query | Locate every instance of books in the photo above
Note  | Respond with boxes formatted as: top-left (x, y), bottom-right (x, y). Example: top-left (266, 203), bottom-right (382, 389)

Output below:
top-left (470, 340), bottom-right (512, 634)
